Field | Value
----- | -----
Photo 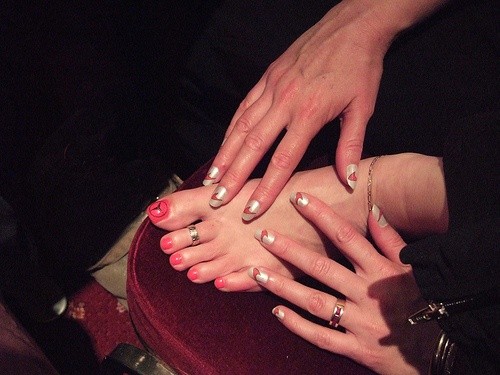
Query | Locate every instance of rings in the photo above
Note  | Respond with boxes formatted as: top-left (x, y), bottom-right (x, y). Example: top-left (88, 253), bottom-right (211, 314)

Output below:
top-left (329, 298), bottom-right (345, 329)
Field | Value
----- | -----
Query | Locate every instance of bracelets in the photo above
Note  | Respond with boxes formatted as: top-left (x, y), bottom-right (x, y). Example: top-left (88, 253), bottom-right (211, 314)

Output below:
top-left (428, 331), bottom-right (456, 375)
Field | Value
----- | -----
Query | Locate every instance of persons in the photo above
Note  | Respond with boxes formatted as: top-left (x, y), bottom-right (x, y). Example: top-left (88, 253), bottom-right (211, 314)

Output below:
top-left (143, 0), bottom-right (498, 375)
